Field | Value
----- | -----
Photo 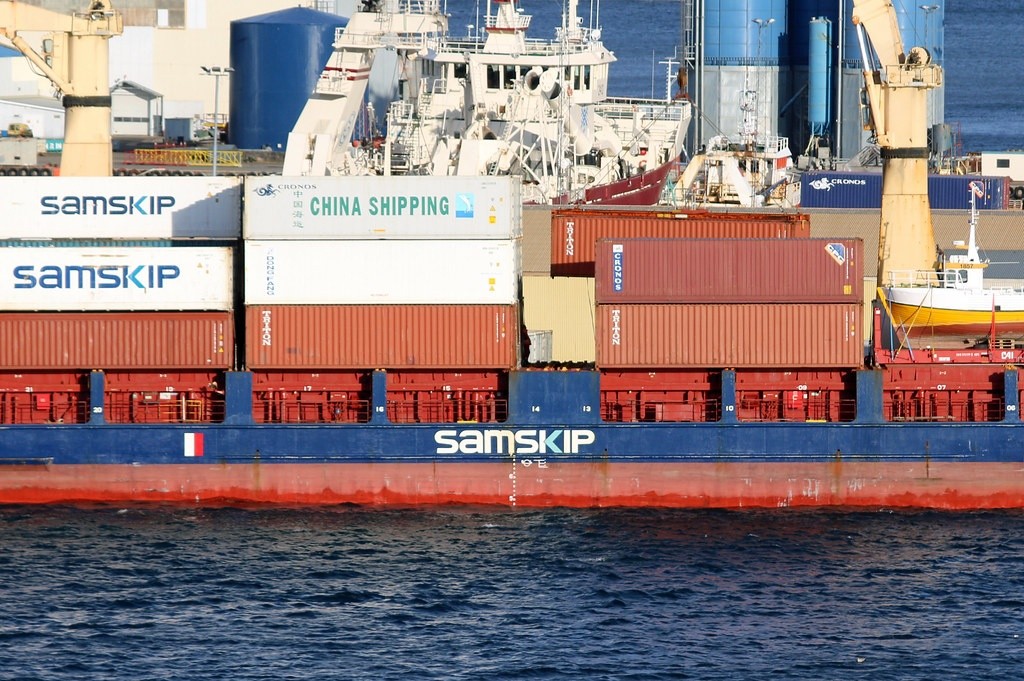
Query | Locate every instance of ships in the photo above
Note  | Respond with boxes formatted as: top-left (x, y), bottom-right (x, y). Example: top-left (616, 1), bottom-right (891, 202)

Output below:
top-left (0, 0), bottom-right (1024, 514)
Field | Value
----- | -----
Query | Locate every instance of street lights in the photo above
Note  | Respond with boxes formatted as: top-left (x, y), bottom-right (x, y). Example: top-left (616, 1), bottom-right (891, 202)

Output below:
top-left (199, 65), bottom-right (236, 176)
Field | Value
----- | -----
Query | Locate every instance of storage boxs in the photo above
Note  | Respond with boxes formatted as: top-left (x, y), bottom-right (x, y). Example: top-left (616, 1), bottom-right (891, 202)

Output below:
top-left (0, 171), bottom-right (1024, 424)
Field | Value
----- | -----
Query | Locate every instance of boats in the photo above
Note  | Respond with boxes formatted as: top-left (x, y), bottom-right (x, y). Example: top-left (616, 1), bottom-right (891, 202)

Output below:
top-left (874, 180), bottom-right (1024, 350)
